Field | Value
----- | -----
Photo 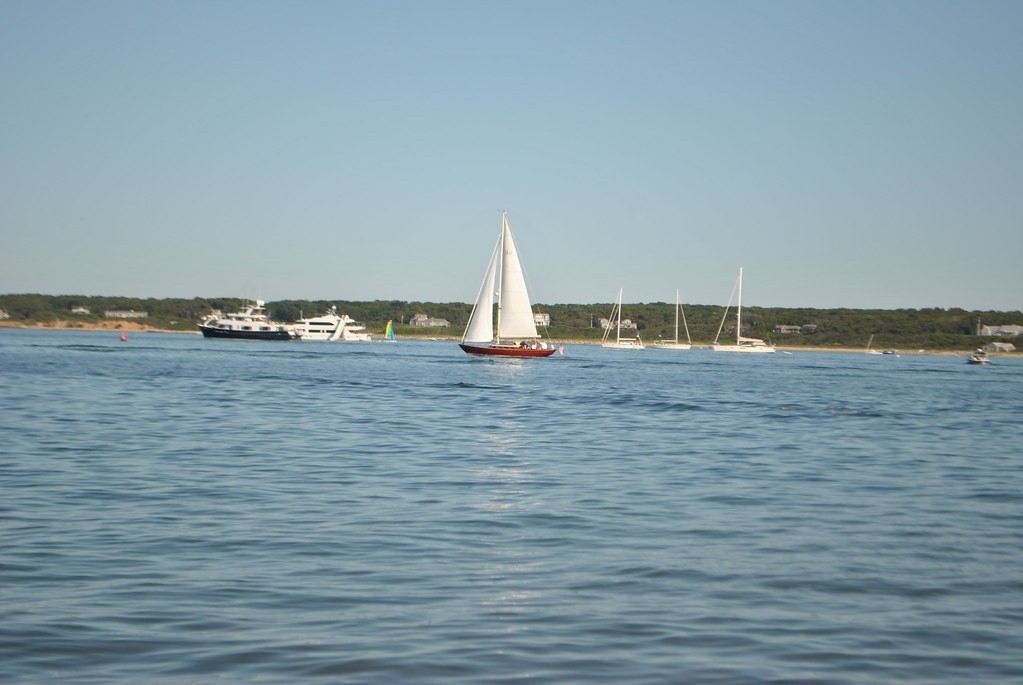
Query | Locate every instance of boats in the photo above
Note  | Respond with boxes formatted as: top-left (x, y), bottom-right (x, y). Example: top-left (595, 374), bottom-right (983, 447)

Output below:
top-left (967, 348), bottom-right (993, 365)
top-left (197, 299), bottom-right (303, 342)
top-left (883, 350), bottom-right (893, 354)
top-left (280, 305), bottom-right (373, 345)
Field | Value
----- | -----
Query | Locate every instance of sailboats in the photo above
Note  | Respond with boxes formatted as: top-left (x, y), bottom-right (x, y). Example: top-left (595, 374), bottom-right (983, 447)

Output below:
top-left (653, 288), bottom-right (692, 350)
top-left (381, 320), bottom-right (397, 343)
top-left (709, 268), bottom-right (777, 354)
top-left (459, 208), bottom-right (556, 357)
top-left (600, 288), bottom-right (646, 351)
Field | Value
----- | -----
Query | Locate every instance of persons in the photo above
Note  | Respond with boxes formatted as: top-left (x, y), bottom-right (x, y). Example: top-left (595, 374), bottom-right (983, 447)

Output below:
top-left (521, 340), bottom-right (531, 349)
